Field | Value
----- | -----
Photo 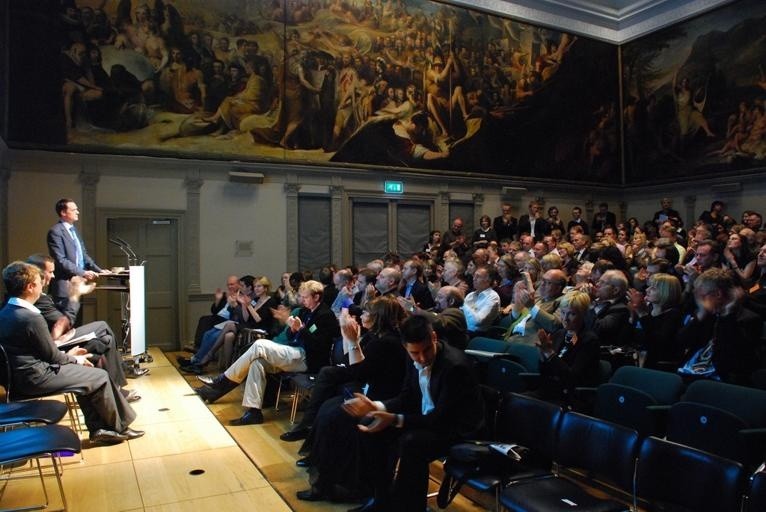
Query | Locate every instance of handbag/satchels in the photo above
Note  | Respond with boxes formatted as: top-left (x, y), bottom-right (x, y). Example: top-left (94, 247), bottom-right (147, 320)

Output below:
top-left (436, 439), bottom-right (532, 509)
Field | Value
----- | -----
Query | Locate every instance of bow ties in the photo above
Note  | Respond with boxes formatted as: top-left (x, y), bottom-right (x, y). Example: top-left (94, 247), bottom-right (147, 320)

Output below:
top-left (574, 252), bottom-right (579, 256)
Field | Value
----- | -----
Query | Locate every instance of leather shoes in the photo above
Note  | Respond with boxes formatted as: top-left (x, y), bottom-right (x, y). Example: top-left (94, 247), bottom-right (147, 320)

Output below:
top-left (348, 495), bottom-right (376, 512)
top-left (125, 366), bottom-right (150, 379)
top-left (93, 428), bottom-right (129, 444)
top-left (279, 424), bottom-right (311, 442)
top-left (229, 410), bottom-right (264, 426)
top-left (296, 457), bottom-right (312, 467)
top-left (296, 489), bottom-right (324, 502)
top-left (118, 427), bottom-right (145, 439)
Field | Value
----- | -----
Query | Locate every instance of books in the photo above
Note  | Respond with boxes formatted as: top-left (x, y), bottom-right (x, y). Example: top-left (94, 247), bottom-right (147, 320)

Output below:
top-left (52, 327), bottom-right (97, 347)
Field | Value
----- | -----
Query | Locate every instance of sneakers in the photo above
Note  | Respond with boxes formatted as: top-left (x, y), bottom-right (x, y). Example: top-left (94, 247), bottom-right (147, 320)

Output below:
top-left (192, 375), bottom-right (218, 404)
top-left (176, 355), bottom-right (192, 367)
top-left (179, 364), bottom-right (209, 376)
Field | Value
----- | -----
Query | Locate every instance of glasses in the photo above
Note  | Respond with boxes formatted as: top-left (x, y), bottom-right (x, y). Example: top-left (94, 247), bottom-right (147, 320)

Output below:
top-left (695, 290), bottom-right (712, 300)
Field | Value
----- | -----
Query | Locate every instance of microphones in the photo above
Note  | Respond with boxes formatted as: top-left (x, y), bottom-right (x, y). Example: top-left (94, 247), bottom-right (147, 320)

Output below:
top-left (117, 236), bottom-right (139, 267)
top-left (106, 239), bottom-right (132, 267)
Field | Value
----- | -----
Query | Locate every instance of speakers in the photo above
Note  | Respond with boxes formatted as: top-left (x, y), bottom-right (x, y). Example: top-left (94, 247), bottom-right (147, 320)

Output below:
top-left (708, 182), bottom-right (741, 192)
top-left (227, 172), bottom-right (269, 183)
top-left (501, 186), bottom-right (529, 198)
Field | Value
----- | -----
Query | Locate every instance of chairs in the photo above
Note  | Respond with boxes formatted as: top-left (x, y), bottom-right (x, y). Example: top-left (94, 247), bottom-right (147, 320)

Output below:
top-left (0, 315), bottom-right (103, 512)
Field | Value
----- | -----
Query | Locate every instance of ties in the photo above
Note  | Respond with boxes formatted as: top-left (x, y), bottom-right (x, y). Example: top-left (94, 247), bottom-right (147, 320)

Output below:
top-left (692, 338), bottom-right (715, 373)
top-left (69, 227), bottom-right (81, 269)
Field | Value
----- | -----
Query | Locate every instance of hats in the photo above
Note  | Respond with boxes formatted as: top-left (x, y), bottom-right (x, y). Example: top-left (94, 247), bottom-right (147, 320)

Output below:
top-left (668, 217), bottom-right (678, 222)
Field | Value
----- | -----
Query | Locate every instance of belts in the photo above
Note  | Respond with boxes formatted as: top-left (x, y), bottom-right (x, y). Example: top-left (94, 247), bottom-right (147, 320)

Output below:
top-left (513, 332), bottom-right (522, 335)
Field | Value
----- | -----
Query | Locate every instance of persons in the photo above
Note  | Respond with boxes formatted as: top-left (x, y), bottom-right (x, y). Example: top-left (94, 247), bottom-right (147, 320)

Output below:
top-left (0, 260), bottom-right (145, 445)
top-left (47, 315), bottom-right (140, 403)
top-left (24, 253), bottom-right (150, 378)
top-left (47, 197), bottom-right (113, 328)
top-left (177, 194), bottom-right (765, 512)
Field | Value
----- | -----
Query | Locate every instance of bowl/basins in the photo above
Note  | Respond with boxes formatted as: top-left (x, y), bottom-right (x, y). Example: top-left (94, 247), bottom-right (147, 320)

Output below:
top-left (112, 267), bottom-right (125, 273)
